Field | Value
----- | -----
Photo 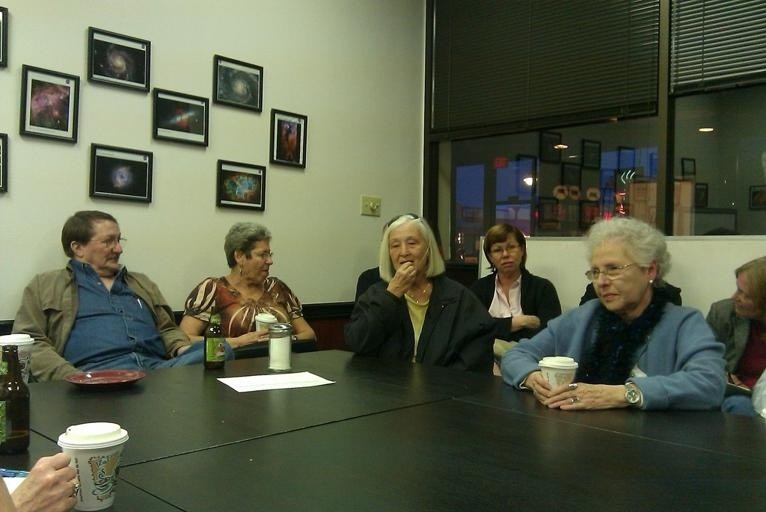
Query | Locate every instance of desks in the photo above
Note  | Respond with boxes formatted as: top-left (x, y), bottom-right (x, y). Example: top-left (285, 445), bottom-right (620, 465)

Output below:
top-left (117, 397), bottom-right (766, 511)
top-left (1, 350), bottom-right (454, 473)
top-left (247, 351), bottom-right (766, 457)
top-left (0, 427), bottom-right (186, 512)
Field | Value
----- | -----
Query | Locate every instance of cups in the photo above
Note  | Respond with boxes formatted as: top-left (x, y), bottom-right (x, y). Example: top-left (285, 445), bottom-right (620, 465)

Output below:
top-left (58, 422), bottom-right (129, 512)
top-left (254, 313), bottom-right (278, 345)
top-left (0, 333), bottom-right (35, 387)
top-left (538, 356), bottom-right (579, 408)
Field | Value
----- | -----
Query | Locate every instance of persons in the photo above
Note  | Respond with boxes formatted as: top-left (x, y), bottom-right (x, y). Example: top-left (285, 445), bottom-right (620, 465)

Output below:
top-left (180, 221), bottom-right (317, 356)
top-left (468, 225), bottom-right (563, 375)
top-left (0, 451), bottom-right (81, 511)
top-left (500, 224), bottom-right (728, 412)
top-left (11, 211), bottom-right (236, 382)
top-left (578, 278), bottom-right (683, 307)
top-left (344, 216), bottom-right (496, 374)
top-left (354, 212), bottom-right (417, 304)
top-left (705, 256), bottom-right (766, 415)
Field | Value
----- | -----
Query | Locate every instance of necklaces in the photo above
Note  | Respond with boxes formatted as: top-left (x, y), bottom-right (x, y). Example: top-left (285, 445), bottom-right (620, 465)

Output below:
top-left (406, 280), bottom-right (430, 305)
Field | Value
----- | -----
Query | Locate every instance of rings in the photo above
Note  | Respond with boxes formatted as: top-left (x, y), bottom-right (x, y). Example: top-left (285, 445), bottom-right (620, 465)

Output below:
top-left (568, 381), bottom-right (579, 391)
top-left (68, 481), bottom-right (81, 490)
top-left (569, 396), bottom-right (581, 405)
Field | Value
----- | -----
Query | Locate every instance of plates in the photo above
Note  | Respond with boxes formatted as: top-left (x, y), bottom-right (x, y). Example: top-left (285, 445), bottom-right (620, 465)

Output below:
top-left (64, 371), bottom-right (145, 389)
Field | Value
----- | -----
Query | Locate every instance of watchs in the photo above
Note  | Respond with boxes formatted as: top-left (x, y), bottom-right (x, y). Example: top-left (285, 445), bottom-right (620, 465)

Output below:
top-left (291, 332), bottom-right (299, 346)
top-left (625, 382), bottom-right (642, 408)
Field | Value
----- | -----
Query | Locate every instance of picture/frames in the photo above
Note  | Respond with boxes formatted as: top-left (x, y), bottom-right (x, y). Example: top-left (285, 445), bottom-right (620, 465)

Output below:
top-left (270, 109), bottom-right (307, 168)
top-left (151, 88), bottom-right (210, 146)
top-left (216, 158), bottom-right (266, 212)
top-left (0, 132), bottom-right (9, 192)
top-left (20, 64), bottom-right (80, 146)
top-left (87, 26), bottom-right (151, 92)
top-left (212, 54), bottom-right (264, 114)
top-left (89, 144), bottom-right (153, 203)
top-left (0, 7), bottom-right (7, 68)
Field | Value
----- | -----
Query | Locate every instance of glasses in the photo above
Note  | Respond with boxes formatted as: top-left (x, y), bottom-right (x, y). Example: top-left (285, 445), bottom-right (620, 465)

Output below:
top-left (252, 252), bottom-right (275, 259)
top-left (92, 238), bottom-right (128, 248)
top-left (584, 262), bottom-right (635, 281)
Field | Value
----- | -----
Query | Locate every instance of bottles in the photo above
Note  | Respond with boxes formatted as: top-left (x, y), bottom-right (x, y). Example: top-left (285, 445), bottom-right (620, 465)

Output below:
top-left (0, 345), bottom-right (30, 455)
top-left (203, 306), bottom-right (225, 370)
